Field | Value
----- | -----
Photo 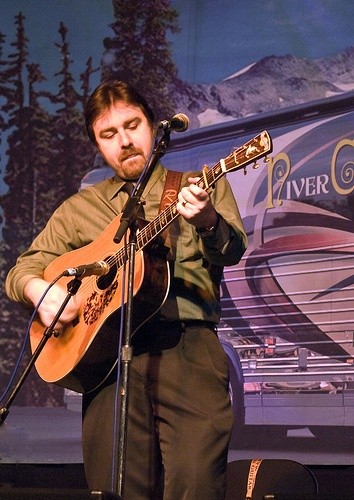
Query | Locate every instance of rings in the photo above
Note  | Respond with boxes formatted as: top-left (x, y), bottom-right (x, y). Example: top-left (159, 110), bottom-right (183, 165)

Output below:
top-left (182, 201), bottom-right (188, 207)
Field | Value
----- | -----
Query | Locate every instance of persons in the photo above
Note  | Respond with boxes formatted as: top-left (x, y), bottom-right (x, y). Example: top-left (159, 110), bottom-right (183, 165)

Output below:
top-left (5, 81), bottom-right (248, 500)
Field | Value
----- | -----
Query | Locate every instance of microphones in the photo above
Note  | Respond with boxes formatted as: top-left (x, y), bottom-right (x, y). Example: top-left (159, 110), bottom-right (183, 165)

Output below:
top-left (62, 260), bottom-right (110, 277)
top-left (157, 113), bottom-right (190, 132)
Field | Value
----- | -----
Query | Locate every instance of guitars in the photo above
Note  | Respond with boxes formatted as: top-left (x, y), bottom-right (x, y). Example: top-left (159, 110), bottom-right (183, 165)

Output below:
top-left (29, 130), bottom-right (273, 393)
